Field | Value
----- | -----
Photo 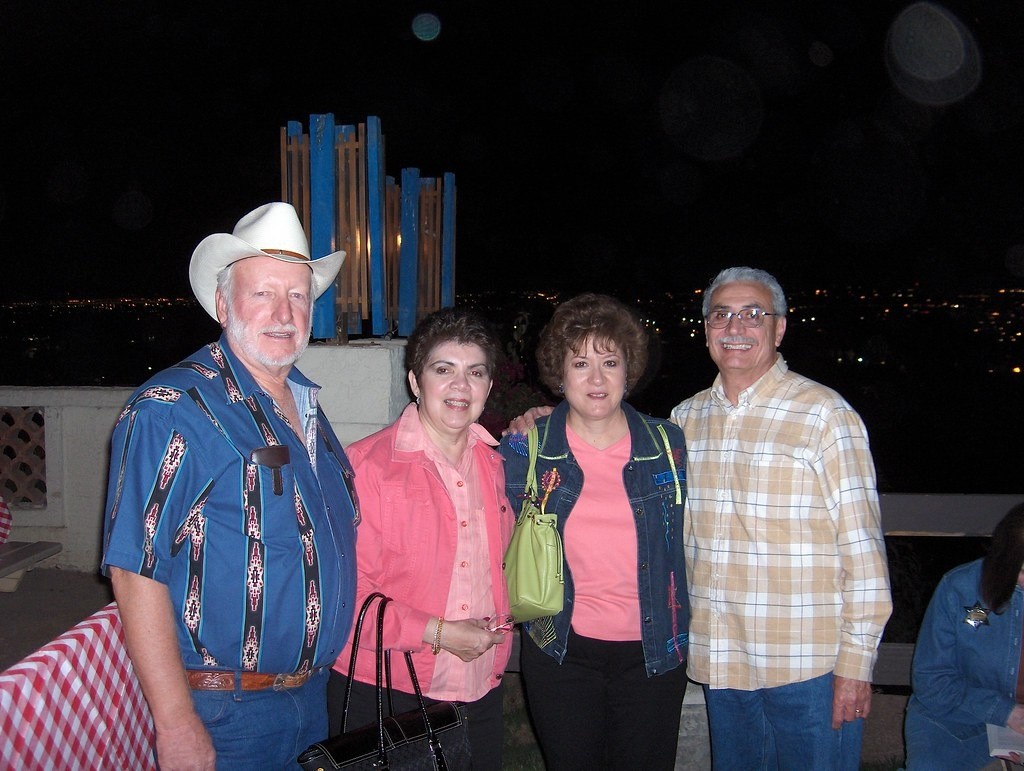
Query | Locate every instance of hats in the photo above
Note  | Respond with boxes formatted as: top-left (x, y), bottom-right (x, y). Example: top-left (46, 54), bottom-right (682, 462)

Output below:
top-left (189, 203), bottom-right (346, 323)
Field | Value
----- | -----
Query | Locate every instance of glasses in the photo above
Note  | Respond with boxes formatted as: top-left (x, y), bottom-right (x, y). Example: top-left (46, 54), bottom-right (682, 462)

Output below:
top-left (704, 310), bottom-right (777, 329)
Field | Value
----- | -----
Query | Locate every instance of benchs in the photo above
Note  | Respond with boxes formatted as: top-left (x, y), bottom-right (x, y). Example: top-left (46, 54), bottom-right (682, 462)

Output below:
top-left (0, 542), bottom-right (63, 595)
top-left (0, 599), bottom-right (159, 771)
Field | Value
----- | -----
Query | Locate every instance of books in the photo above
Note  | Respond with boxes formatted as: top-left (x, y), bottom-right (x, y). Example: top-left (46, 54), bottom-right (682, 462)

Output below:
top-left (986, 721), bottom-right (1024, 757)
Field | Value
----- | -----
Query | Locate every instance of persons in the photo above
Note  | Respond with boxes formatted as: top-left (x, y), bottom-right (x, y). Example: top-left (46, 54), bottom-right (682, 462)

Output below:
top-left (326, 308), bottom-right (518, 771)
top-left (99, 201), bottom-right (363, 771)
top-left (496, 295), bottom-right (688, 771)
top-left (901, 504), bottom-right (1024, 771)
top-left (496, 265), bottom-right (894, 771)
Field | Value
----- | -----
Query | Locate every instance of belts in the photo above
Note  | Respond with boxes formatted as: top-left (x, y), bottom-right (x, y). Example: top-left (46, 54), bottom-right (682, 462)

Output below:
top-left (187, 670), bottom-right (312, 690)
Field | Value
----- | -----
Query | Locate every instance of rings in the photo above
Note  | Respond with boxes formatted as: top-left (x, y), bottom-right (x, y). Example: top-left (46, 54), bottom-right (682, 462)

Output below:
top-left (854, 708), bottom-right (864, 713)
top-left (514, 418), bottom-right (516, 420)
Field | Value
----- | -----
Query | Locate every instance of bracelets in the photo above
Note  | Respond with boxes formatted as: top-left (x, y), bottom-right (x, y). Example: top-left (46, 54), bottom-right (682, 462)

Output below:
top-left (431, 615), bottom-right (445, 656)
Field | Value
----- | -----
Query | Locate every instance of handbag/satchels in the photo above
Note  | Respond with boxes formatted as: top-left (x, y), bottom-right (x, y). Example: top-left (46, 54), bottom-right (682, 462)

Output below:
top-left (298, 593), bottom-right (470, 771)
top-left (504, 426), bottom-right (563, 622)
